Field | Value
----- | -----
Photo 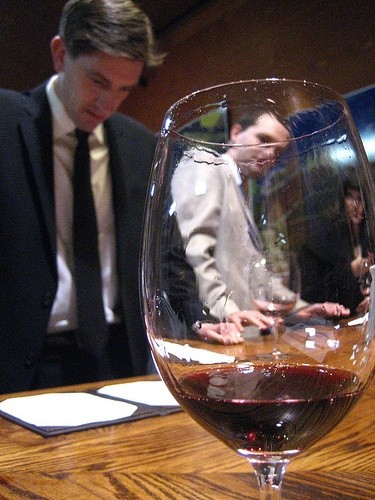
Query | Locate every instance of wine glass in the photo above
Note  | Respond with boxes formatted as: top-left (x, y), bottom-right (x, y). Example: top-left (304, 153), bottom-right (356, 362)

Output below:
top-left (248, 254), bottom-right (299, 344)
top-left (141, 77), bottom-right (375, 500)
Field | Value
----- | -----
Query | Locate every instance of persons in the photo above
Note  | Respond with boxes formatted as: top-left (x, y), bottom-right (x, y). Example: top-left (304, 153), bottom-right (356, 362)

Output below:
top-left (170, 108), bottom-right (350, 332)
top-left (0, 0), bottom-right (244, 394)
top-left (288, 179), bottom-right (375, 320)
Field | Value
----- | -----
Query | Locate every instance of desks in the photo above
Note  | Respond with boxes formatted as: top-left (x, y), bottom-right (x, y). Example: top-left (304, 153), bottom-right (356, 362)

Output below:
top-left (0, 317), bottom-right (375, 500)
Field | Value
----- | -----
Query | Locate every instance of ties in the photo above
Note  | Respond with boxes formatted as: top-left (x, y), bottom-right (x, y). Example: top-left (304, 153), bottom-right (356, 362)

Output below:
top-left (235, 173), bottom-right (264, 255)
top-left (72, 127), bottom-right (110, 356)
